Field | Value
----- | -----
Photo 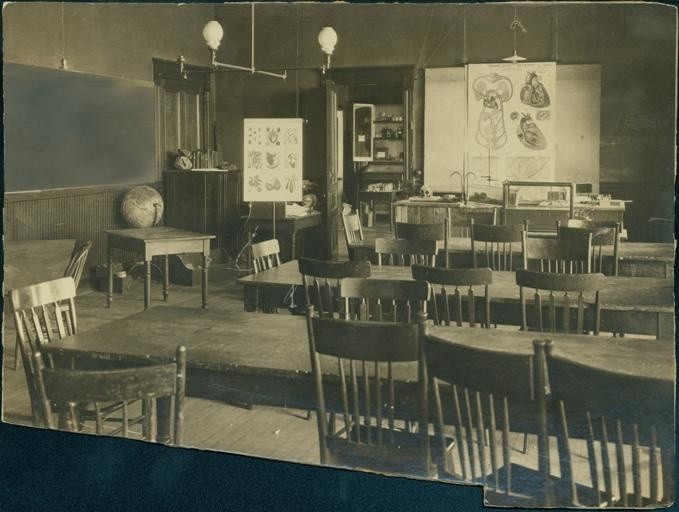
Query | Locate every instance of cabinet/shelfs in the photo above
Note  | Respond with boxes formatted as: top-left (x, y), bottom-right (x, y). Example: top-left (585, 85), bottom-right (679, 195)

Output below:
top-left (347, 94), bottom-right (407, 210)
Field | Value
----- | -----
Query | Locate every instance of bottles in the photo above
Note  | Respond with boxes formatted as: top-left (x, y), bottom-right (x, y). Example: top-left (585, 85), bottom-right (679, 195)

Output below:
top-left (374, 111), bottom-right (403, 139)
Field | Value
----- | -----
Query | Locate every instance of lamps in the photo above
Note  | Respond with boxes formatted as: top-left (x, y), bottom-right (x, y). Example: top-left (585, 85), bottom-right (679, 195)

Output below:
top-left (316, 25), bottom-right (339, 75)
top-left (201, 20), bottom-right (223, 61)
